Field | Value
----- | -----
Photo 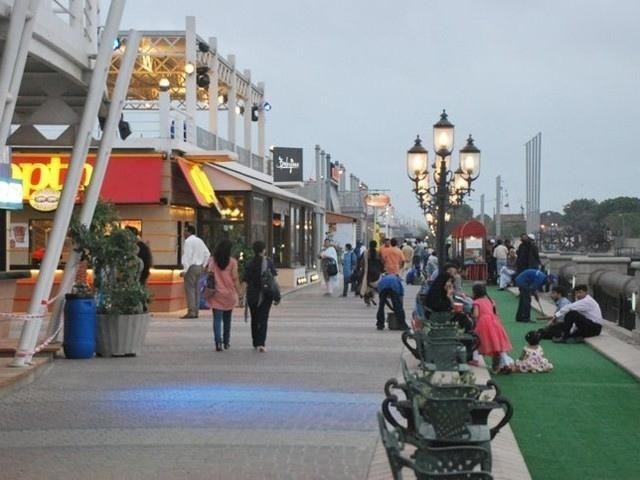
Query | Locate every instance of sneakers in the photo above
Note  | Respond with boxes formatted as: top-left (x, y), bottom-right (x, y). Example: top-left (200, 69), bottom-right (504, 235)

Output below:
top-left (254, 344), bottom-right (266, 353)
top-left (216, 342), bottom-right (231, 351)
top-left (179, 314), bottom-right (200, 319)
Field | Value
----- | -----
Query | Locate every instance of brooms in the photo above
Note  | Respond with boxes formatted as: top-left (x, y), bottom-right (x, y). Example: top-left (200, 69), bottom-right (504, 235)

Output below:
top-left (533, 294), bottom-right (555, 321)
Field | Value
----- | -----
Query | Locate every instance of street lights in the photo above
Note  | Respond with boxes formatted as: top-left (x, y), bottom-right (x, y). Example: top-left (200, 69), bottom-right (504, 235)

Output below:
top-left (538, 224), bottom-right (545, 250)
top-left (402, 103), bottom-right (483, 274)
top-left (550, 223), bottom-right (558, 250)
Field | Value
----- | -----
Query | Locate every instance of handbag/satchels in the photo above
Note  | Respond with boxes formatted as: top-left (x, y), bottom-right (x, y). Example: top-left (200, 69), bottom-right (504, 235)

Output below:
top-left (387, 312), bottom-right (405, 330)
top-left (198, 255), bottom-right (216, 299)
top-left (260, 255), bottom-right (281, 303)
top-left (326, 258), bottom-right (338, 276)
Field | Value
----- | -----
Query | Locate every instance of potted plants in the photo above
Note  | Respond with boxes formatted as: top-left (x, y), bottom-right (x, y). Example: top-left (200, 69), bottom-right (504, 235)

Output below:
top-left (71, 184), bottom-right (155, 357)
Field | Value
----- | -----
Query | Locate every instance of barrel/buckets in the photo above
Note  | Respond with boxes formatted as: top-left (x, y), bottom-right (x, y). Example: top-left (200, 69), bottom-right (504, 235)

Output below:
top-left (63, 293), bottom-right (97, 359)
top-left (386, 312), bottom-right (400, 330)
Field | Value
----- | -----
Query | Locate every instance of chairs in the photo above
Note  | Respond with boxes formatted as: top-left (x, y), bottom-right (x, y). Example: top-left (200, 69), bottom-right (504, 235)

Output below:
top-left (377, 294), bottom-right (513, 480)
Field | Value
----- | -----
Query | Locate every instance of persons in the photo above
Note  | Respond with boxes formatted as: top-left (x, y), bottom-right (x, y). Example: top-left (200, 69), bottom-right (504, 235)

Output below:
top-left (317, 234), bottom-right (603, 373)
top-left (179, 225), bottom-right (211, 318)
top-left (207, 239), bottom-right (244, 351)
top-left (125, 225), bottom-right (151, 288)
top-left (241, 241), bottom-right (281, 353)
